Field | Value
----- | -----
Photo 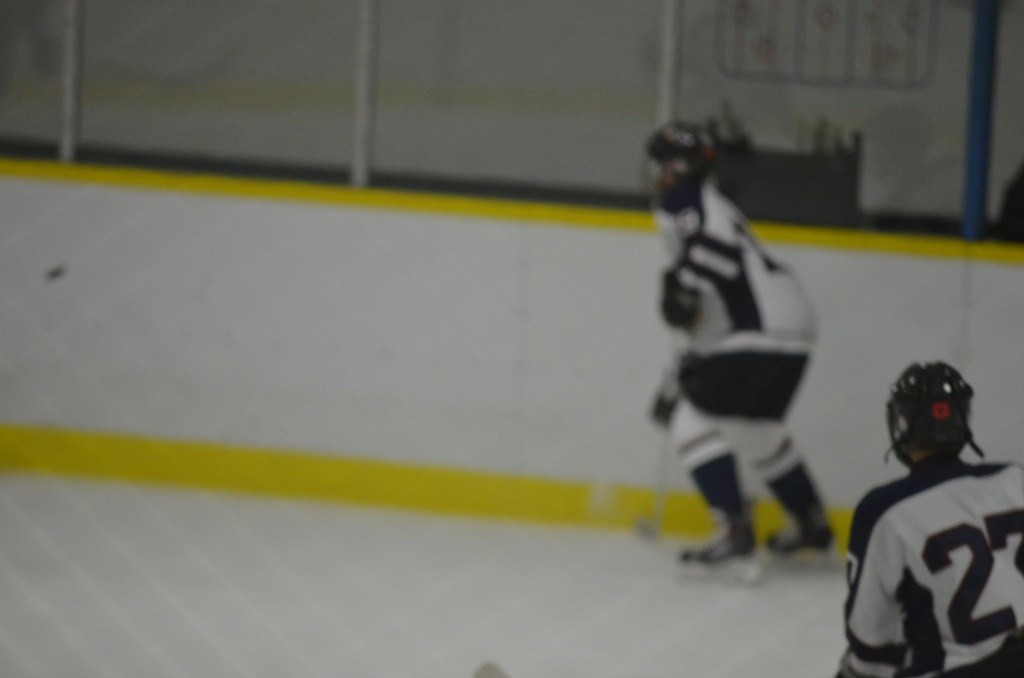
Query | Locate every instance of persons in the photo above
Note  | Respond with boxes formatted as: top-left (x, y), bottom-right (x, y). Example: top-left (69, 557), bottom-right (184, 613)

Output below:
top-left (833, 360), bottom-right (1024, 678)
top-left (644, 120), bottom-right (836, 586)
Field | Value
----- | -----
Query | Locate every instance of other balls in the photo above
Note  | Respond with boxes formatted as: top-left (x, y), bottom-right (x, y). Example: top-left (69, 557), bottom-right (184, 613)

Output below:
top-left (46, 267), bottom-right (64, 279)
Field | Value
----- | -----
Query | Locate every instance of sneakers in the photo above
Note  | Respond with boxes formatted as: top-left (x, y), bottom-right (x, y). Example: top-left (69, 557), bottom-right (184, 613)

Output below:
top-left (763, 524), bottom-right (834, 569)
top-left (676, 523), bottom-right (758, 581)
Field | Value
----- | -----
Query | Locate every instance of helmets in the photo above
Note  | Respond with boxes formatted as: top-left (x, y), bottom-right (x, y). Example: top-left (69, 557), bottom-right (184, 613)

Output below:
top-left (644, 122), bottom-right (729, 214)
top-left (888, 361), bottom-right (973, 468)
